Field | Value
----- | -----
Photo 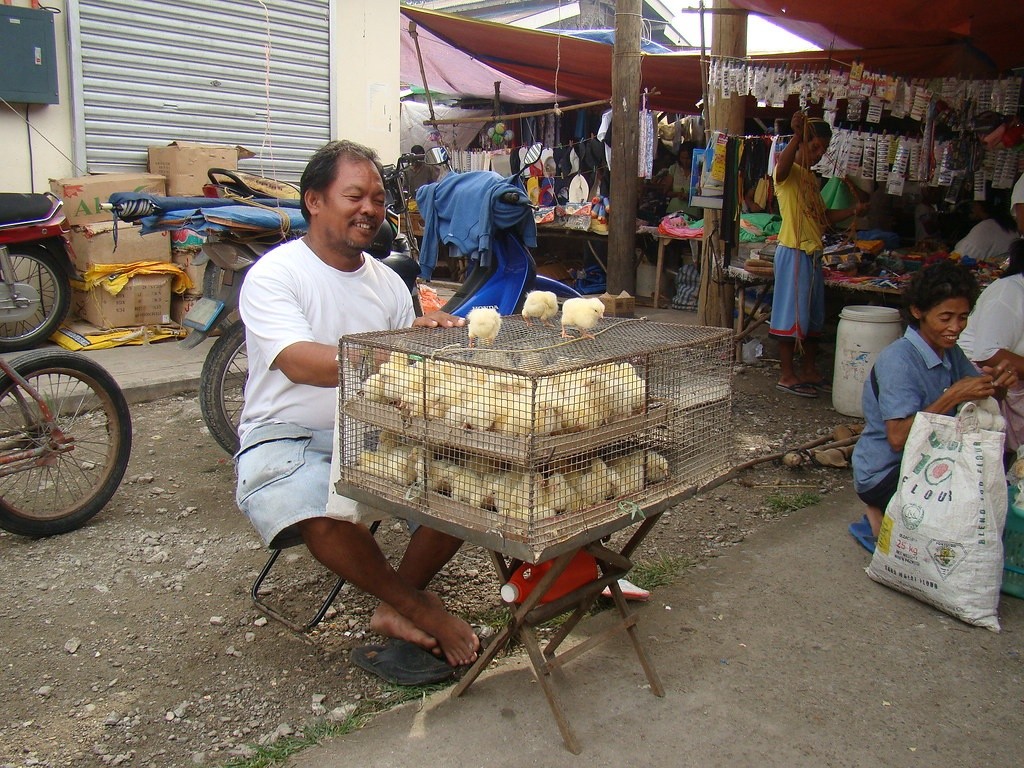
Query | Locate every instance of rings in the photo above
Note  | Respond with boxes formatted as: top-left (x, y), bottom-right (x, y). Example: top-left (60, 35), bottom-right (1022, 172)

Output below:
top-left (1006, 369), bottom-right (1013, 374)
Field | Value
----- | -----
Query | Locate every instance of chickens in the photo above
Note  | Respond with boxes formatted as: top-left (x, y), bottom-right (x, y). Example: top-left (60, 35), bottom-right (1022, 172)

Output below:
top-left (356, 291), bottom-right (668, 523)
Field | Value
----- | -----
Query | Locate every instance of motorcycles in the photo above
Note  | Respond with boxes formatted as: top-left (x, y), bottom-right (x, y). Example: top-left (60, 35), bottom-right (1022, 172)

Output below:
top-left (0, 337), bottom-right (135, 542)
top-left (0, 192), bottom-right (86, 356)
top-left (177, 148), bottom-right (586, 459)
top-left (201, 143), bottom-right (451, 306)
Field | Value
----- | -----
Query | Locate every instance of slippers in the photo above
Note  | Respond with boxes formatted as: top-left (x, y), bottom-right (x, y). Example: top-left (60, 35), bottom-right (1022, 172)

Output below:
top-left (351, 640), bottom-right (453, 687)
top-left (776, 377), bottom-right (832, 398)
top-left (848, 514), bottom-right (878, 555)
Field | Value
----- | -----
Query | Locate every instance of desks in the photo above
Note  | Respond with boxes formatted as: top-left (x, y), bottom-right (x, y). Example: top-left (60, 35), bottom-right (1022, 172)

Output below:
top-left (537, 227), bottom-right (703, 309)
top-left (733, 280), bottom-right (908, 364)
top-left (452, 511), bottom-right (666, 756)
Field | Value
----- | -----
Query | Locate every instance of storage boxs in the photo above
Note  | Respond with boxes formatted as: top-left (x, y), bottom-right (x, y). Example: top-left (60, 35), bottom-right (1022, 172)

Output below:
top-left (49, 141), bottom-right (257, 331)
top-left (599, 294), bottom-right (635, 318)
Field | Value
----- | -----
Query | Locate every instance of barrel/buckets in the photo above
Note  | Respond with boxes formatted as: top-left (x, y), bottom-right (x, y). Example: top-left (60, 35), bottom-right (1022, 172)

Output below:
top-left (635, 258), bottom-right (667, 298)
top-left (832, 305), bottom-right (905, 419)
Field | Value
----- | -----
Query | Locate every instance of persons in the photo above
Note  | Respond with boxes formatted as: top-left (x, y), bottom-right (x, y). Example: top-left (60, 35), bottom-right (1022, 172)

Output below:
top-left (770, 110), bottom-right (870, 397)
top-left (943, 172), bottom-right (1024, 554)
top-left (403, 145), bottom-right (437, 200)
top-left (237, 140), bottom-right (478, 667)
top-left (846, 263), bottom-right (1024, 554)
top-left (662, 148), bottom-right (704, 221)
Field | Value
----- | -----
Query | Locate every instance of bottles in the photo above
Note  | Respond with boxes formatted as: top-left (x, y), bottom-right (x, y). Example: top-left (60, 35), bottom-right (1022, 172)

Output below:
top-left (501, 547), bottom-right (598, 605)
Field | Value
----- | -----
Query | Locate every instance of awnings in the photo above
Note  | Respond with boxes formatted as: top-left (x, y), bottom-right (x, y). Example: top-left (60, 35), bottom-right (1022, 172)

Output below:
top-left (400, 0), bottom-right (1024, 113)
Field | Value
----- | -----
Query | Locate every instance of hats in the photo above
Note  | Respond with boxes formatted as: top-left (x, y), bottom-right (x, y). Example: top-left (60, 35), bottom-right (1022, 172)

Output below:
top-left (510, 142), bottom-right (605, 206)
